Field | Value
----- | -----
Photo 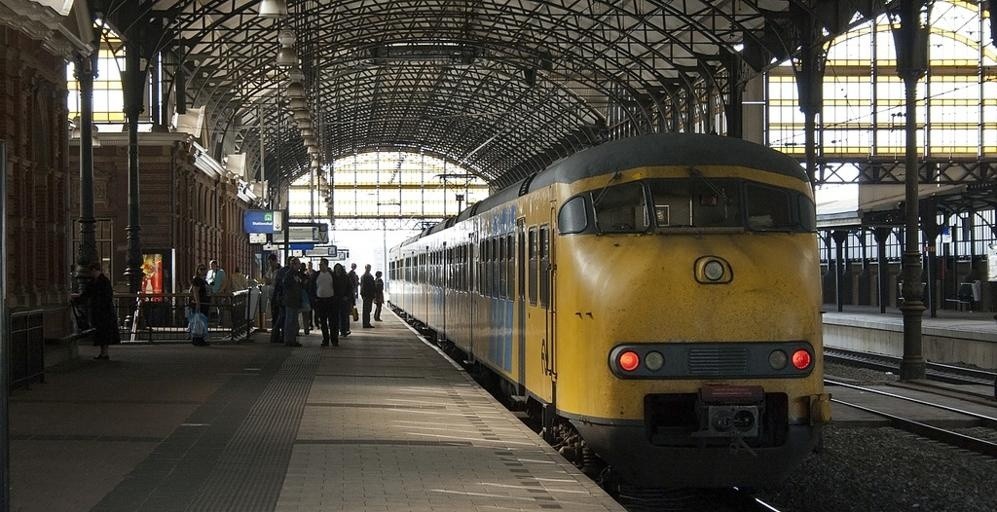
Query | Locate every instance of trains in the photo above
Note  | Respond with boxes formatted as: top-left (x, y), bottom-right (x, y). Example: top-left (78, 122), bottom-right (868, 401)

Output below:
top-left (388, 132), bottom-right (833, 488)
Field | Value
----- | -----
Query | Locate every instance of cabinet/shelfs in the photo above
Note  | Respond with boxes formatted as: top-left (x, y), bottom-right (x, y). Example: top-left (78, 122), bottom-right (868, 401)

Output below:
top-left (6, 306), bottom-right (45, 396)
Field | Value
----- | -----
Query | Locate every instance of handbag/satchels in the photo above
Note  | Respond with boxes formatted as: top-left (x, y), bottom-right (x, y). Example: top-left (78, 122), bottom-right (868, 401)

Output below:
top-left (185, 310), bottom-right (208, 339)
top-left (352, 305), bottom-right (359, 321)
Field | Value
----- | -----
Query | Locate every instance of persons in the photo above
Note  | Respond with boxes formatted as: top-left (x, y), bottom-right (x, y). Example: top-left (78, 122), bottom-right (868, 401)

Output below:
top-left (179, 252), bottom-right (389, 346)
top-left (73, 261), bottom-right (123, 360)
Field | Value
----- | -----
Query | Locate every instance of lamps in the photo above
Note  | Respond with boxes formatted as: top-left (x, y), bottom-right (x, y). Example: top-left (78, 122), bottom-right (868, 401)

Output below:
top-left (287, 69), bottom-right (323, 168)
top-left (257, 1), bottom-right (288, 18)
top-left (276, 29), bottom-right (299, 67)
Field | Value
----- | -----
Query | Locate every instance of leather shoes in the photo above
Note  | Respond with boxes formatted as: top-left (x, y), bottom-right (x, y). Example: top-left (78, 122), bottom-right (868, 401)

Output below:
top-left (333, 341), bottom-right (338, 346)
top-left (94, 354), bottom-right (109, 360)
top-left (321, 341), bottom-right (329, 346)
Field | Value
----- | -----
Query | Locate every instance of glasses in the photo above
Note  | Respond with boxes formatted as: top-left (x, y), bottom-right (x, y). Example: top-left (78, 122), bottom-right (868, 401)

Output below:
top-left (200, 267), bottom-right (206, 271)
